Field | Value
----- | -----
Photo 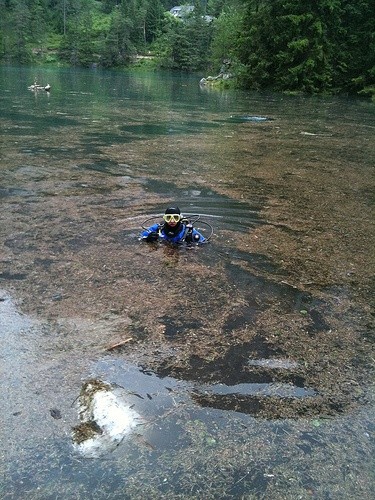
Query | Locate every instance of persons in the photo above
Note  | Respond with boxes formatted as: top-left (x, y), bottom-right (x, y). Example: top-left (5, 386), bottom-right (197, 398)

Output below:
top-left (139, 207), bottom-right (207, 247)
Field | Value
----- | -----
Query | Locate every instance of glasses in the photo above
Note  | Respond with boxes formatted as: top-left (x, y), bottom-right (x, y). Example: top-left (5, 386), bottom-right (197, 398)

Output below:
top-left (161, 213), bottom-right (181, 223)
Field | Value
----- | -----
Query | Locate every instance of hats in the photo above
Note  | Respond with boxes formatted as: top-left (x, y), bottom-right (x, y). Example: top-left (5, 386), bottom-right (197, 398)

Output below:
top-left (165, 207), bottom-right (180, 215)
